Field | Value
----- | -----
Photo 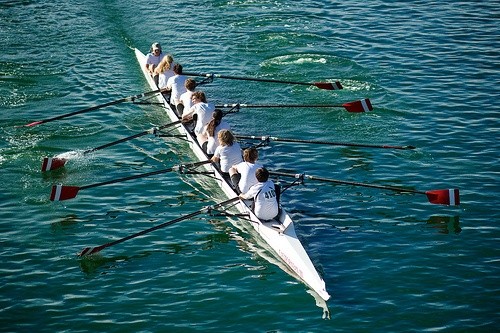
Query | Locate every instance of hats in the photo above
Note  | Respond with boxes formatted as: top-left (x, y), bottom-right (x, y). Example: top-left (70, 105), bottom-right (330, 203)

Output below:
top-left (152, 42), bottom-right (161, 49)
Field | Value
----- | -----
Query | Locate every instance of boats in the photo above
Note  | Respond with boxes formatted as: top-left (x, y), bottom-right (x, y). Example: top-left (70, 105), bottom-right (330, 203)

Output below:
top-left (133, 47), bottom-right (331, 300)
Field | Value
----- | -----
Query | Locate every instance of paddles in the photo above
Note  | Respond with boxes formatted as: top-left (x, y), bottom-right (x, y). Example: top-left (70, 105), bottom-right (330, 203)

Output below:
top-left (235, 135), bottom-right (417, 152)
top-left (41, 119), bottom-right (182, 172)
top-left (214, 97), bottom-right (374, 113)
top-left (179, 70), bottom-right (344, 91)
top-left (24, 89), bottom-right (159, 128)
top-left (261, 169), bottom-right (460, 206)
top-left (49, 159), bottom-right (212, 202)
top-left (78, 195), bottom-right (241, 260)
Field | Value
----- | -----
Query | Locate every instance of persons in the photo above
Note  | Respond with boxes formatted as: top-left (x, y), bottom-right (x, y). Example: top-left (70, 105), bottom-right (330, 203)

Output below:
top-left (160, 63), bottom-right (189, 111)
top-left (182, 91), bottom-right (215, 139)
top-left (177, 78), bottom-right (197, 118)
top-left (196, 108), bottom-right (231, 159)
top-left (210, 129), bottom-right (244, 179)
top-left (153, 55), bottom-right (175, 89)
top-left (145, 42), bottom-right (164, 77)
top-left (239, 167), bottom-right (278, 222)
top-left (229, 147), bottom-right (263, 194)
